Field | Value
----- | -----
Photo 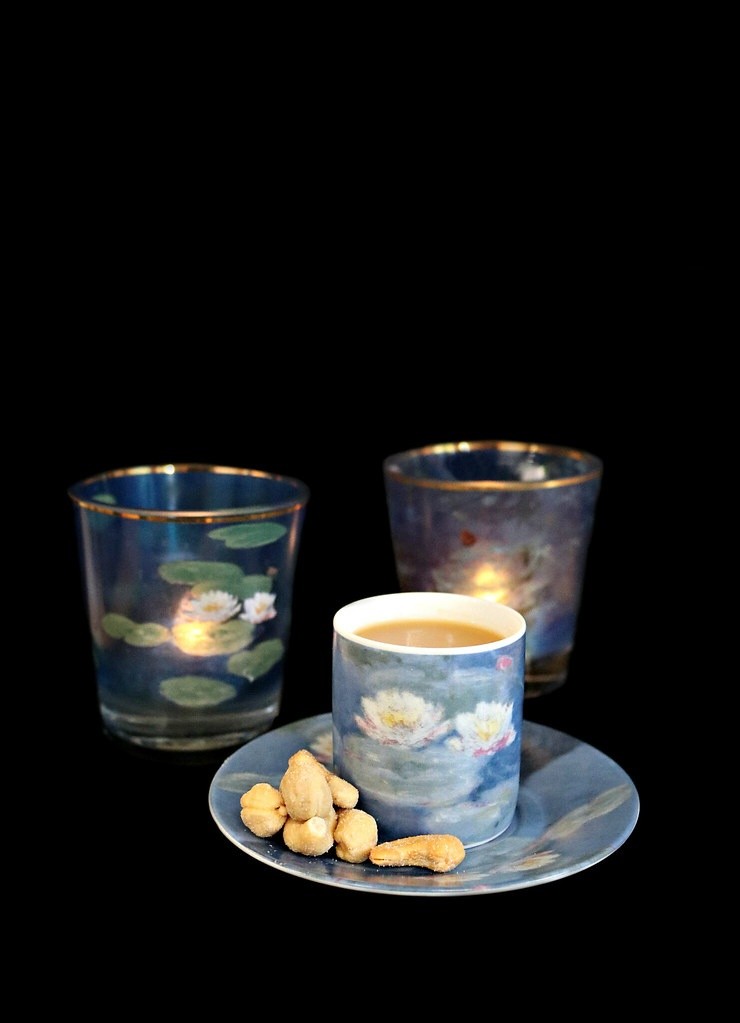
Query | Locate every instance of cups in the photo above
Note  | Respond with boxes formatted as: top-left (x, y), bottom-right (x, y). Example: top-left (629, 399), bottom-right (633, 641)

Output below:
top-left (333, 592), bottom-right (527, 849)
top-left (385, 438), bottom-right (604, 700)
top-left (67, 459), bottom-right (309, 752)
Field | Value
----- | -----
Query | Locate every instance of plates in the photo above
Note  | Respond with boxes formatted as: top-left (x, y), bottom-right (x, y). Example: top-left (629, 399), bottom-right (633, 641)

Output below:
top-left (208, 712), bottom-right (641, 896)
